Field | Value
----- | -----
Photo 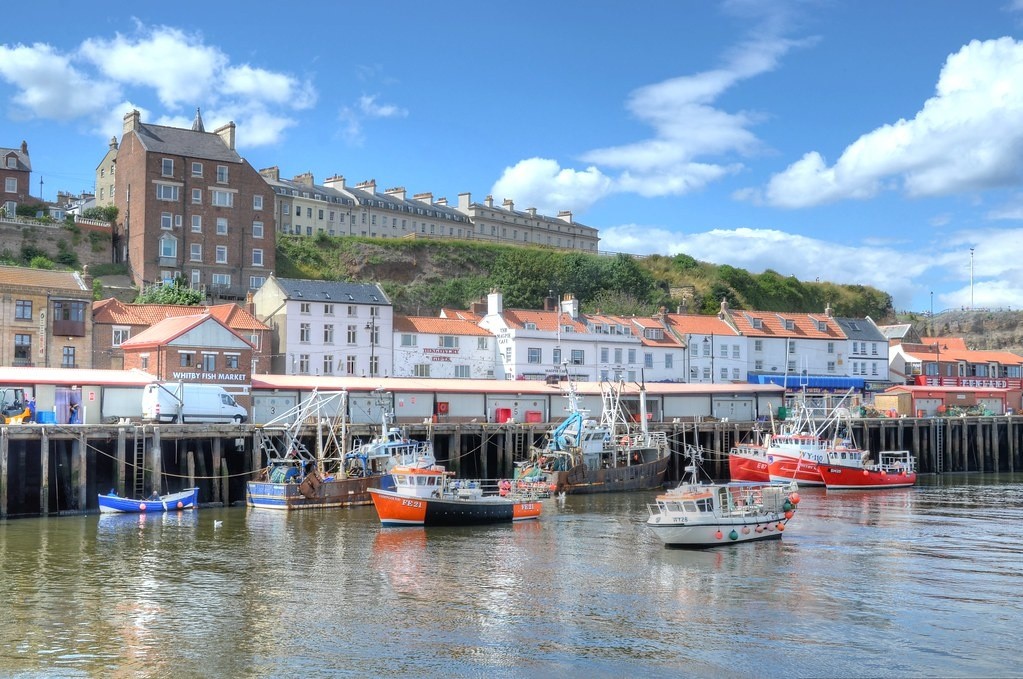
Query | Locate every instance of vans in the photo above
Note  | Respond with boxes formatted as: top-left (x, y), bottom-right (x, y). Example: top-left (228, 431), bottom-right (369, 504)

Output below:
top-left (141, 382), bottom-right (248, 424)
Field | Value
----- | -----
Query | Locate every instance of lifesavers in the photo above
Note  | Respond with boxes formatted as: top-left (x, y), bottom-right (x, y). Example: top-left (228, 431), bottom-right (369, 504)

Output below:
top-left (757, 505), bottom-right (768, 516)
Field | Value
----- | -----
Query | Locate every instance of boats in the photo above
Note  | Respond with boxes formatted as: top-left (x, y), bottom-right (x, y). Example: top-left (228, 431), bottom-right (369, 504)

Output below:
top-left (98, 486), bottom-right (200, 515)
top-left (646, 449), bottom-right (800, 549)
top-left (728, 356), bottom-right (877, 489)
top-left (814, 447), bottom-right (916, 491)
top-left (364, 455), bottom-right (547, 527)
top-left (514, 374), bottom-right (674, 497)
top-left (244, 386), bottom-right (434, 509)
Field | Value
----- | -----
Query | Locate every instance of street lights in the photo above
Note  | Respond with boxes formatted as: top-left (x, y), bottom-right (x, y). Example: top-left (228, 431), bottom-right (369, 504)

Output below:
top-left (364, 317), bottom-right (373, 379)
top-left (936, 341), bottom-right (948, 386)
top-left (702, 333), bottom-right (714, 417)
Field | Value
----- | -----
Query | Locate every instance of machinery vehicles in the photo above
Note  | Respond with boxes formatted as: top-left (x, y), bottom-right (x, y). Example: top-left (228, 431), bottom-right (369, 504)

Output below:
top-left (0, 387), bottom-right (32, 425)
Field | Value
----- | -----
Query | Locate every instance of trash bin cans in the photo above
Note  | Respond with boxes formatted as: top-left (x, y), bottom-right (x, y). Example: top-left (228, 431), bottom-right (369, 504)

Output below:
top-left (433, 413), bottom-right (438, 423)
top-left (526, 411), bottom-right (542, 422)
top-left (778, 406), bottom-right (787, 420)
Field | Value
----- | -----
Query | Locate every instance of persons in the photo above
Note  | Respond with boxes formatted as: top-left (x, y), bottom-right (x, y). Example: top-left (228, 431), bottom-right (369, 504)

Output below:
top-left (29, 397), bottom-right (35, 422)
top-left (69, 403), bottom-right (78, 424)
